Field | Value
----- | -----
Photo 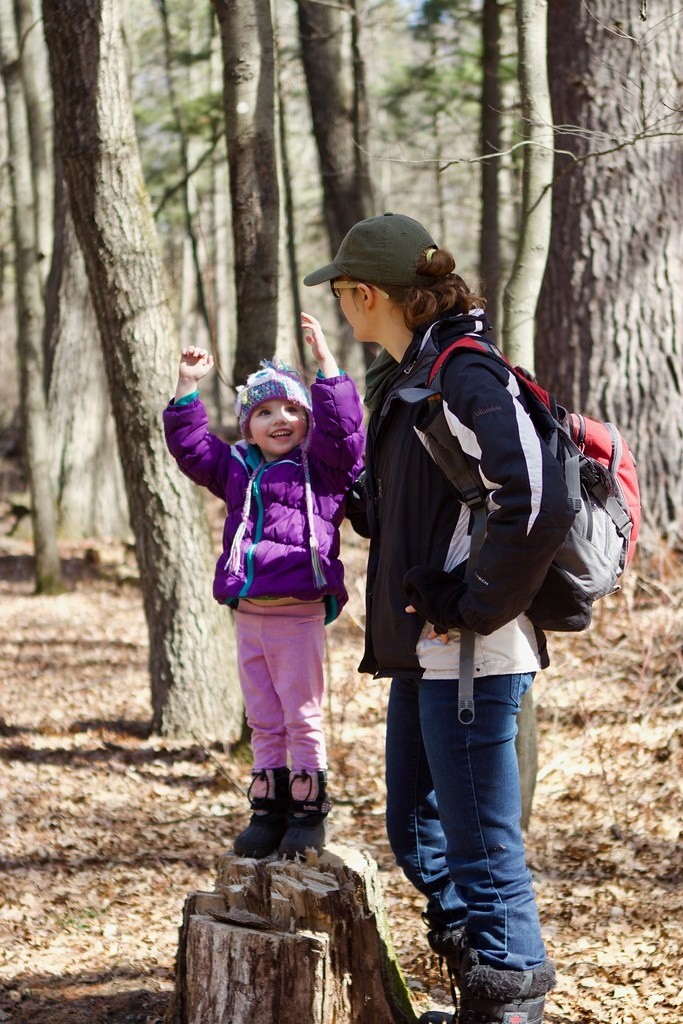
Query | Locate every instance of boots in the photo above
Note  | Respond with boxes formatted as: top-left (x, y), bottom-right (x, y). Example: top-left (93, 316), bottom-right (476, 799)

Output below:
top-left (462, 962), bottom-right (557, 1024)
top-left (418, 926), bottom-right (471, 1024)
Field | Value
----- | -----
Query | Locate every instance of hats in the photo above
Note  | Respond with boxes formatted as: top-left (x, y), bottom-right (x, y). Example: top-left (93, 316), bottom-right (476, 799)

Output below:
top-left (224, 356), bottom-right (328, 589)
top-left (303, 211), bottom-right (439, 287)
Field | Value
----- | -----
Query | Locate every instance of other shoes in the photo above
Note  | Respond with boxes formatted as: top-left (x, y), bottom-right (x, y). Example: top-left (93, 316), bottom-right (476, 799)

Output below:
top-left (278, 818), bottom-right (326, 863)
top-left (233, 813), bottom-right (287, 858)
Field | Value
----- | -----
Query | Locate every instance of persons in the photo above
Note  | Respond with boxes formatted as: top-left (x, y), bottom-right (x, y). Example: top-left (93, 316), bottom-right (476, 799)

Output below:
top-left (304, 212), bottom-right (578, 1024)
top-left (163, 312), bottom-right (366, 861)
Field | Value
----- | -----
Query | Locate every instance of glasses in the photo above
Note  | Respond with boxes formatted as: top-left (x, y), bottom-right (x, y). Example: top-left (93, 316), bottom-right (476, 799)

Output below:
top-left (328, 278), bottom-right (390, 300)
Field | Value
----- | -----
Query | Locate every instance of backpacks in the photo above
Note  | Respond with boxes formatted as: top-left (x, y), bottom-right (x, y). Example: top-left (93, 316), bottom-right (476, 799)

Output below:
top-left (421, 333), bottom-right (642, 633)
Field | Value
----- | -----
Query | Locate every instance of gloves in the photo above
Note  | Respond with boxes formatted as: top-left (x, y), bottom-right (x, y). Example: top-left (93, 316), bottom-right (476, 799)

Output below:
top-left (402, 565), bottom-right (467, 628)
top-left (343, 484), bottom-right (371, 540)
